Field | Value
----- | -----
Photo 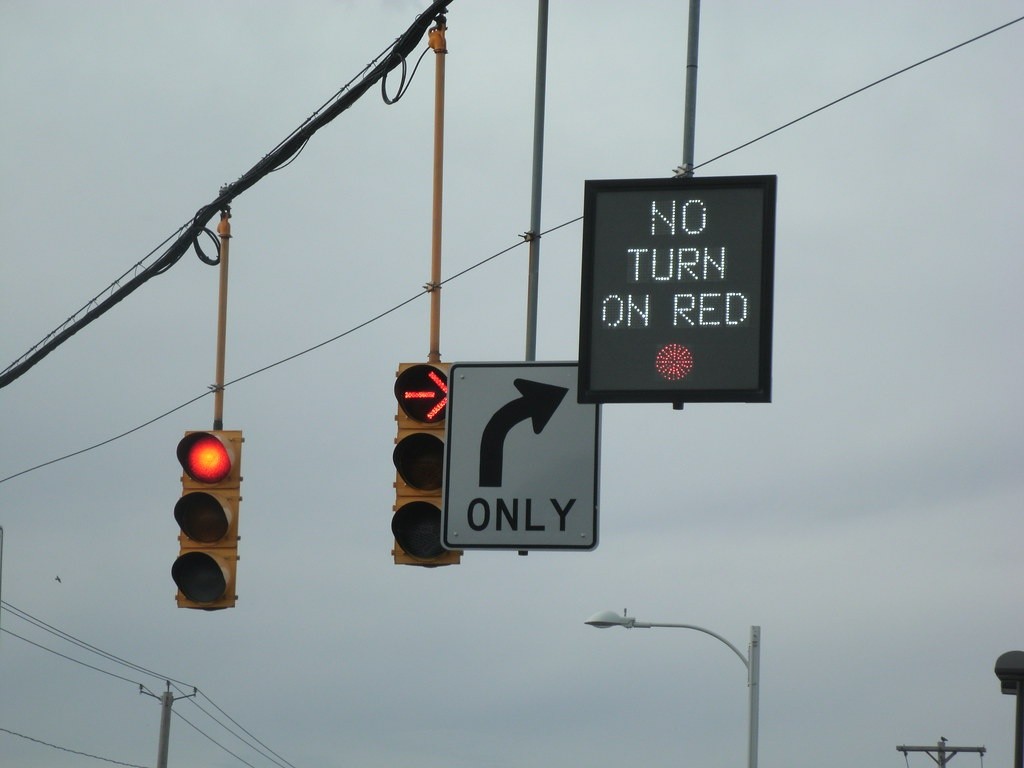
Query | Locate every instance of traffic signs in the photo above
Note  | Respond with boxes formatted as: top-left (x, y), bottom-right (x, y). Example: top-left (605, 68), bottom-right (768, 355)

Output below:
top-left (441, 360), bottom-right (602, 552)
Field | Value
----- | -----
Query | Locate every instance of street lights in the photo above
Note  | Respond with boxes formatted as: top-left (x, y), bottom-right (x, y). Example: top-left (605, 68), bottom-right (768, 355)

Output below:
top-left (583, 611), bottom-right (760, 768)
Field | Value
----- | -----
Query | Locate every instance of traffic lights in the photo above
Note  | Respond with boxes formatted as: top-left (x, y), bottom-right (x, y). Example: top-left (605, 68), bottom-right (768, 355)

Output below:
top-left (391, 363), bottom-right (465, 567)
top-left (175, 430), bottom-right (243, 612)
